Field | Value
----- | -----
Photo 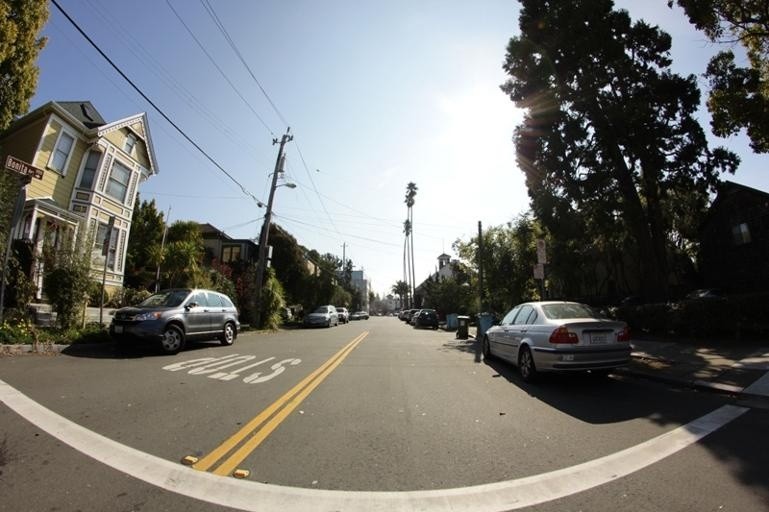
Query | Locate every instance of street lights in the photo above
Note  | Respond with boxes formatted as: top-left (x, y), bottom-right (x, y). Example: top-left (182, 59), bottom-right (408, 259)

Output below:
top-left (246, 180), bottom-right (297, 329)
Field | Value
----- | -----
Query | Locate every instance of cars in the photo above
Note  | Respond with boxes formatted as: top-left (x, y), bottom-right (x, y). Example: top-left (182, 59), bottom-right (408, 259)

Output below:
top-left (482, 299), bottom-right (633, 386)
top-left (398, 308), bottom-right (420, 324)
top-left (349, 311), bottom-right (370, 322)
top-left (304, 304), bottom-right (338, 328)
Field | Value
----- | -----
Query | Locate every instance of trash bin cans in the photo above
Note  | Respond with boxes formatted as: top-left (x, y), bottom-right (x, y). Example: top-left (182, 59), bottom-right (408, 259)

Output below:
top-left (456, 315), bottom-right (470, 339)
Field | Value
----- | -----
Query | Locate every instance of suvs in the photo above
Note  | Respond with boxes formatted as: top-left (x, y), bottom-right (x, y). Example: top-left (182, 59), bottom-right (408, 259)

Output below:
top-left (415, 309), bottom-right (440, 329)
top-left (102, 285), bottom-right (243, 356)
top-left (333, 307), bottom-right (349, 323)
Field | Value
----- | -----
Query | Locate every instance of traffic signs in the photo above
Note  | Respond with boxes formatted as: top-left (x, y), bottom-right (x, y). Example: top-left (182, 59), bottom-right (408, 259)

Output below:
top-left (5, 155), bottom-right (44, 180)
